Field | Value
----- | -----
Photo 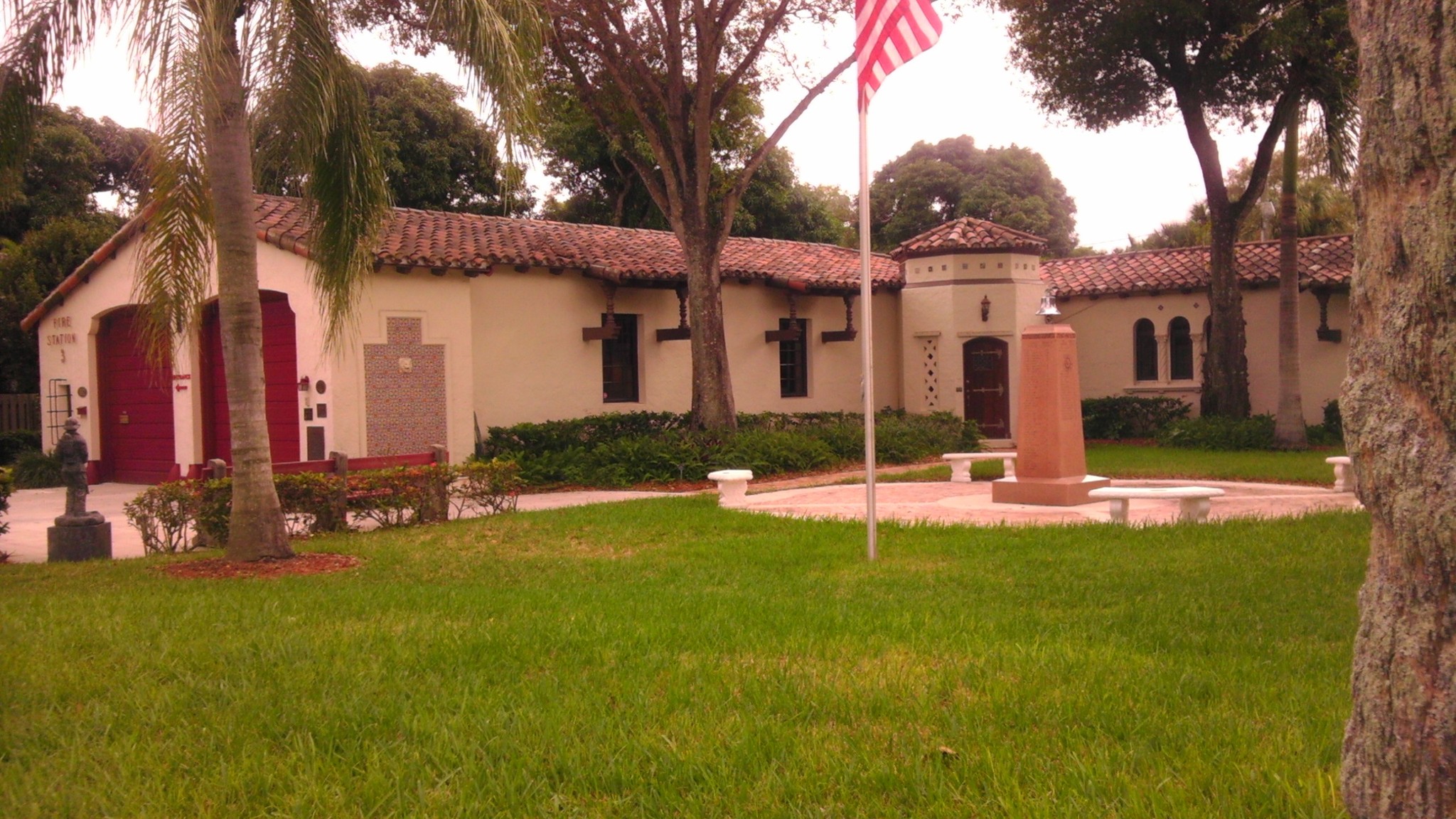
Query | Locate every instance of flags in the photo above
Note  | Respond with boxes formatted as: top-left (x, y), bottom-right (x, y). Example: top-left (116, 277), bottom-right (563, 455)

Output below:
top-left (853, 2), bottom-right (944, 108)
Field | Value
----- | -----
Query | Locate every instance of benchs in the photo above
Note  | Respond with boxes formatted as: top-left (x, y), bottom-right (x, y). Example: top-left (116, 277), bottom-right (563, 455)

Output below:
top-left (946, 448), bottom-right (1021, 485)
top-left (1092, 484), bottom-right (1225, 525)
top-left (199, 442), bottom-right (453, 537)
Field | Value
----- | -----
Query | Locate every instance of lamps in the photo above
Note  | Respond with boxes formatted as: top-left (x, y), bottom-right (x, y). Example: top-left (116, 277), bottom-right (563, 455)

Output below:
top-left (980, 294), bottom-right (993, 319)
